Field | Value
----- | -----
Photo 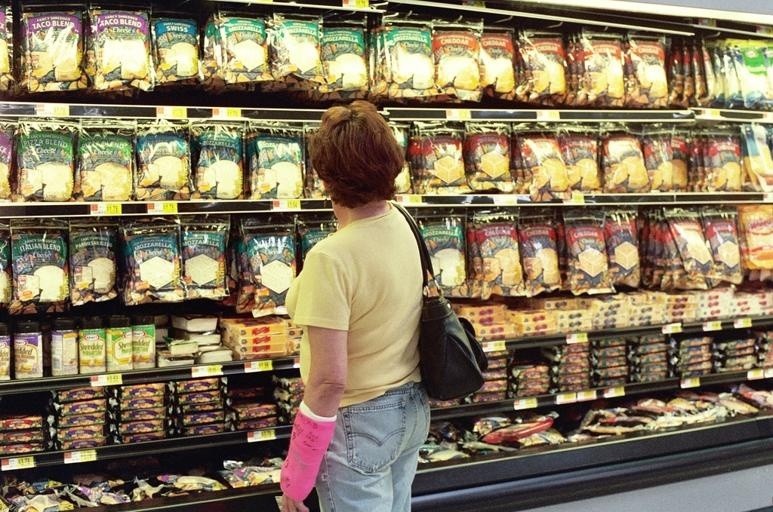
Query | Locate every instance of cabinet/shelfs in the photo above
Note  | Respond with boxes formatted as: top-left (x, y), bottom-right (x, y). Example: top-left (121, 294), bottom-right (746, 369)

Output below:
top-left (0, 292), bottom-right (769, 386)
top-left (2, 125), bottom-right (768, 204)
top-left (50, 414), bottom-right (768, 512)
top-left (0, 0), bottom-right (769, 121)
top-left (0, 325), bottom-right (769, 474)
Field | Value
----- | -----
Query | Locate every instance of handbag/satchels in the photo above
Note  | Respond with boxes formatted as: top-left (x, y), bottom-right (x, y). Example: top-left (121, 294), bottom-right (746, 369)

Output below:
top-left (393, 201), bottom-right (488, 401)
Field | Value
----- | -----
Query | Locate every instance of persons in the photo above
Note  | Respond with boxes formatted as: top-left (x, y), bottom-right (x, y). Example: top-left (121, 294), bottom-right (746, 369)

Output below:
top-left (278, 99), bottom-right (433, 512)
